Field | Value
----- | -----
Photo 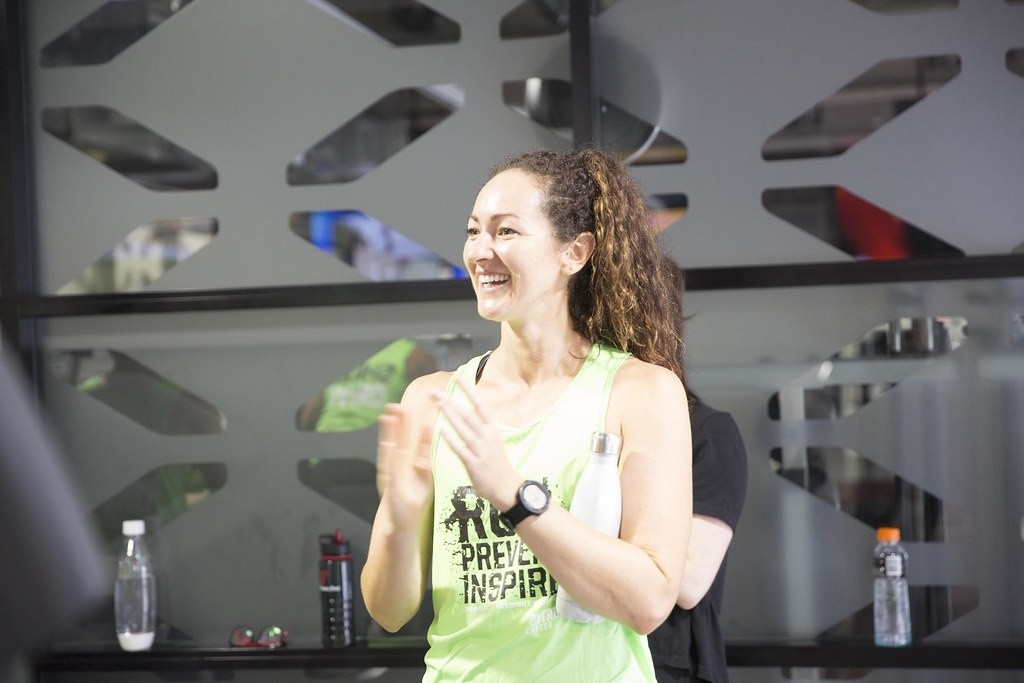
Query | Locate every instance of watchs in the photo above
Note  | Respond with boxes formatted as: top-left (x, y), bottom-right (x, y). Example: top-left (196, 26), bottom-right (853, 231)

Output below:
top-left (499, 479), bottom-right (552, 528)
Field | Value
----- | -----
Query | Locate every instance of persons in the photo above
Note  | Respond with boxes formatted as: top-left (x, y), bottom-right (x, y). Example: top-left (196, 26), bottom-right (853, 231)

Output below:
top-left (604, 253), bottom-right (750, 683)
top-left (355, 148), bottom-right (700, 683)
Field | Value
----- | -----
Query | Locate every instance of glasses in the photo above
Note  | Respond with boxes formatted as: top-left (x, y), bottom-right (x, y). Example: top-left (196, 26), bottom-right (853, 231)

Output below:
top-left (228, 624), bottom-right (290, 648)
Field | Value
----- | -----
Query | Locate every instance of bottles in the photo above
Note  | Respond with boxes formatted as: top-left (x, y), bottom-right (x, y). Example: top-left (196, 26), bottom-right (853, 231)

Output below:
top-left (114, 520), bottom-right (157, 652)
top-left (871, 526), bottom-right (913, 648)
top-left (319, 528), bottom-right (355, 649)
